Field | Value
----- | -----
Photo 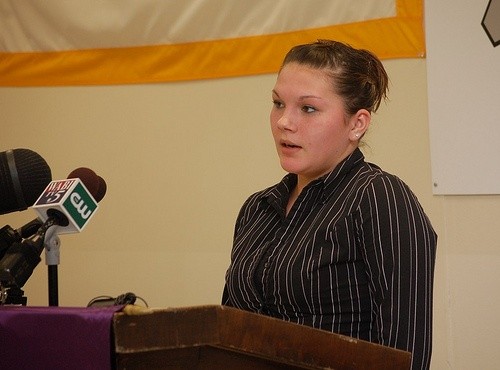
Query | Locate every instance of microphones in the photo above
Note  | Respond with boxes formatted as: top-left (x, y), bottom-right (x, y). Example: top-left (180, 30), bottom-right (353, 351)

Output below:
top-left (0, 148), bottom-right (107, 290)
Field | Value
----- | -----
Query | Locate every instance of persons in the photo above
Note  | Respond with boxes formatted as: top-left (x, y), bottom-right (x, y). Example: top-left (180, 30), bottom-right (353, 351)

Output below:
top-left (222, 38), bottom-right (438, 370)
